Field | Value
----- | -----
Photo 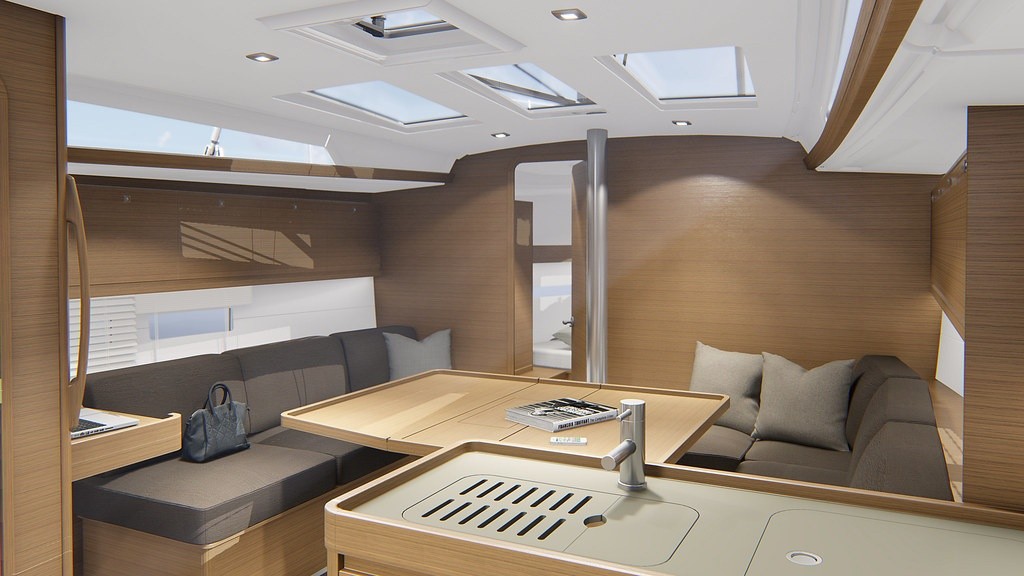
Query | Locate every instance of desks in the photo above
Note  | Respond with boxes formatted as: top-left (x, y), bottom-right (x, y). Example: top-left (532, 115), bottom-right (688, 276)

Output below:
top-left (71, 409), bottom-right (183, 482)
top-left (279, 368), bottom-right (732, 465)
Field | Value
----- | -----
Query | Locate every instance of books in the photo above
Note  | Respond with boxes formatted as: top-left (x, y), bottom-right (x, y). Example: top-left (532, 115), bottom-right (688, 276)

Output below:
top-left (505, 397), bottom-right (620, 433)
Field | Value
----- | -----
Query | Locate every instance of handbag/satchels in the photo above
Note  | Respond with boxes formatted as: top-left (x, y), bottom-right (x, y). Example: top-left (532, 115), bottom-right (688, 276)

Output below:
top-left (182, 383), bottom-right (250, 463)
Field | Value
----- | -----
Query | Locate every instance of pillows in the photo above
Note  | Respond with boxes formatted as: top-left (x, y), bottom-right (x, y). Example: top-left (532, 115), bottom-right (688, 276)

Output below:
top-left (688, 340), bottom-right (764, 436)
top-left (382, 329), bottom-right (451, 382)
top-left (751, 350), bottom-right (857, 452)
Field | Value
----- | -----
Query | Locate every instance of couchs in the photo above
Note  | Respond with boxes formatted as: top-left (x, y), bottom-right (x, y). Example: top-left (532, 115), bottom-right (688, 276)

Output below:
top-left (326, 326), bottom-right (419, 398)
top-left (676, 354), bottom-right (955, 504)
top-left (220, 333), bottom-right (411, 486)
top-left (71, 351), bottom-right (336, 545)
